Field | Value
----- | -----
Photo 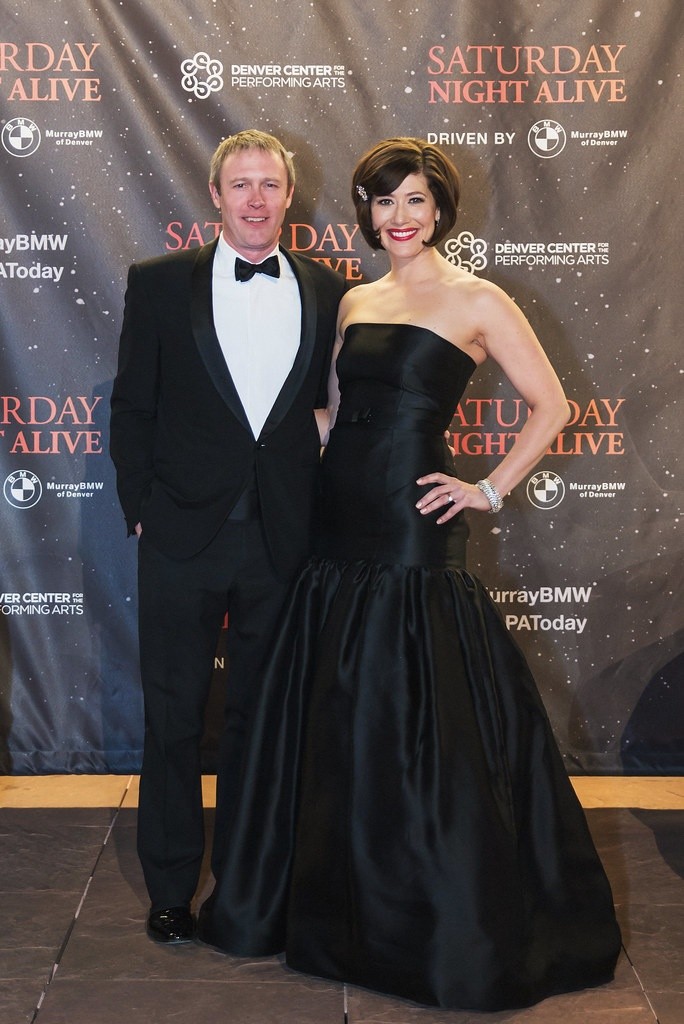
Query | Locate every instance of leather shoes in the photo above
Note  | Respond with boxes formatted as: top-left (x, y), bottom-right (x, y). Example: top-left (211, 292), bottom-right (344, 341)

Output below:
top-left (147, 905), bottom-right (193, 944)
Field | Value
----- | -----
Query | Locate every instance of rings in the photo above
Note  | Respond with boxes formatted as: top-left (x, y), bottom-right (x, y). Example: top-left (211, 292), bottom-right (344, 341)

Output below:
top-left (446, 492), bottom-right (453, 501)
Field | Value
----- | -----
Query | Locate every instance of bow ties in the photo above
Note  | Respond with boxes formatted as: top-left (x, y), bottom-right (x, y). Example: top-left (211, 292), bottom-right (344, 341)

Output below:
top-left (235, 255), bottom-right (280, 282)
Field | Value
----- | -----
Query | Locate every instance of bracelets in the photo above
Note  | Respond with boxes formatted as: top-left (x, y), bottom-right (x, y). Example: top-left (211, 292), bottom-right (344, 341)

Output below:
top-left (476, 478), bottom-right (504, 514)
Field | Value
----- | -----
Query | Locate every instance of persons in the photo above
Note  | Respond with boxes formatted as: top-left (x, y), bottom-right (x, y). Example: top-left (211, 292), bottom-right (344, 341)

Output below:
top-left (111, 128), bottom-right (455, 945)
top-left (199, 134), bottom-right (622, 1014)
